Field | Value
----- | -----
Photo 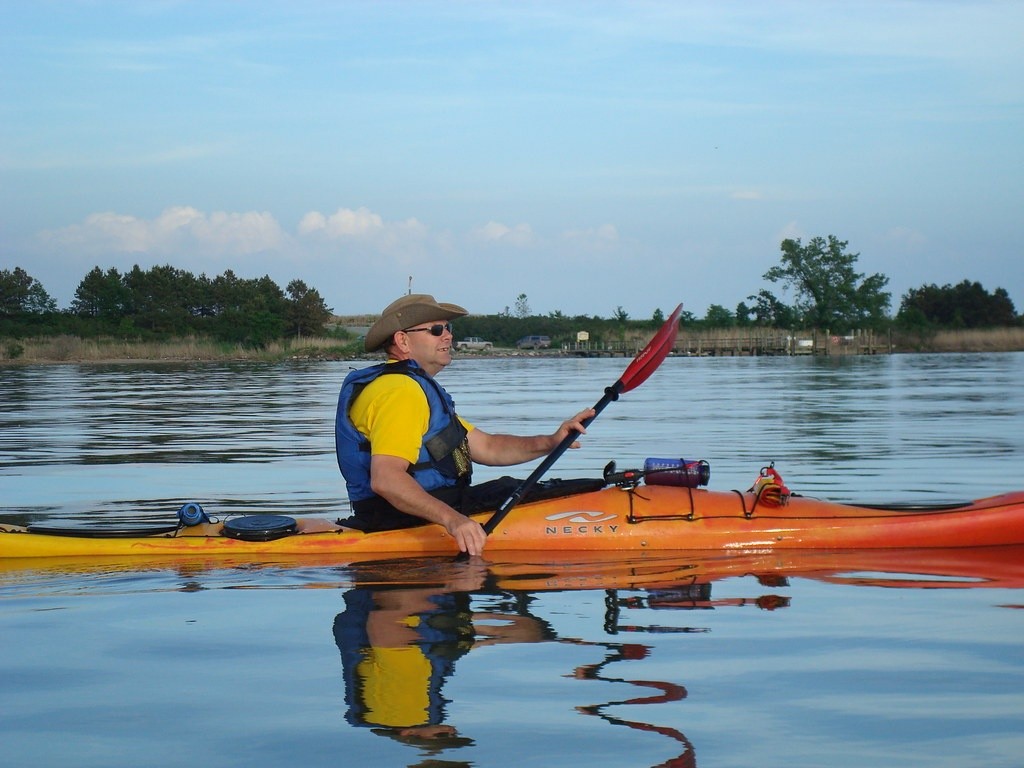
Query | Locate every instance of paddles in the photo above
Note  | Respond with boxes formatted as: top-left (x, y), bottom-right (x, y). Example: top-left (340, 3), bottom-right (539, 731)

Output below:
top-left (456, 302), bottom-right (684, 559)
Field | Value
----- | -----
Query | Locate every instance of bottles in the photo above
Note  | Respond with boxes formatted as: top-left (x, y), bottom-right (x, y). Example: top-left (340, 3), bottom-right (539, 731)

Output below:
top-left (643, 457), bottom-right (711, 489)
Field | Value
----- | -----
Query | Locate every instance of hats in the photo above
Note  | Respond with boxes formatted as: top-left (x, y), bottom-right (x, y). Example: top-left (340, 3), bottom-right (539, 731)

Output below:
top-left (370, 729), bottom-right (475, 750)
top-left (365, 294), bottom-right (469, 352)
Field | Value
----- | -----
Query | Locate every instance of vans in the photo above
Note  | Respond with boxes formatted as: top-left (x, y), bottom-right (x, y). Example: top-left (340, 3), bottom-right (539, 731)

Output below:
top-left (516, 336), bottom-right (551, 350)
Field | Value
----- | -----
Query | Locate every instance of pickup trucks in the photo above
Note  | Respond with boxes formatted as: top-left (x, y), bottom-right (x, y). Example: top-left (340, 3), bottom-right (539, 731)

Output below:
top-left (452, 337), bottom-right (493, 351)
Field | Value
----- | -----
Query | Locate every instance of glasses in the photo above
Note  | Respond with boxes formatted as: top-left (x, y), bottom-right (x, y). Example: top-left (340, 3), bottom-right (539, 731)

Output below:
top-left (391, 323), bottom-right (452, 340)
top-left (423, 733), bottom-right (459, 739)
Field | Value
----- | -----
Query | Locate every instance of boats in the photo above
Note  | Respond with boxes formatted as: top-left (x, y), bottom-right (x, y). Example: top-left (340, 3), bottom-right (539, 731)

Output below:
top-left (0, 482), bottom-right (1024, 565)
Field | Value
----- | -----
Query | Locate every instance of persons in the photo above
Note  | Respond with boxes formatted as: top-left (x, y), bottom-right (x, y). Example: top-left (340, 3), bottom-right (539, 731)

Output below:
top-left (335, 294), bottom-right (595, 556)
